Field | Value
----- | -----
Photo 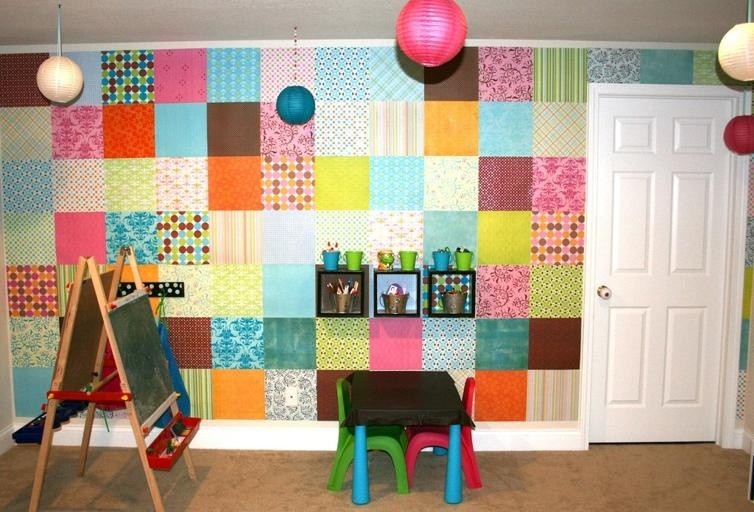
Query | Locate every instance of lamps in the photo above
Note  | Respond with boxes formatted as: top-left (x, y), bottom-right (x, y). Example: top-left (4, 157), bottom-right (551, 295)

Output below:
top-left (275, 25), bottom-right (315, 126)
top-left (35, 4), bottom-right (85, 105)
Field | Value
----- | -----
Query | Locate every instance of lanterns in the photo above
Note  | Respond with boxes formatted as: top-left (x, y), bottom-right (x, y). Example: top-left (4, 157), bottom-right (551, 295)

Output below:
top-left (36, 55), bottom-right (84, 103)
top-left (276, 85), bottom-right (315, 125)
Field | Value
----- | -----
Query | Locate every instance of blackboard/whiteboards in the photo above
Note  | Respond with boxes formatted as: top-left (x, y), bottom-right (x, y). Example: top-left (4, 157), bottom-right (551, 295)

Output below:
top-left (101, 286), bottom-right (180, 438)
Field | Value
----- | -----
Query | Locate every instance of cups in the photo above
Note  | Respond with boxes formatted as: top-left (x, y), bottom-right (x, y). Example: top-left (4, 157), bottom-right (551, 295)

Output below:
top-left (454, 251), bottom-right (473, 271)
top-left (319, 251), bottom-right (339, 271)
top-left (379, 293), bottom-right (409, 314)
top-left (329, 294), bottom-right (354, 313)
top-left (397, 250), bottom-right (419, 270)
top-left (432, 250), bottom-right (451, 271)
top-left (438, 294), bottom-right (467, 314)
top-left (341, 251), bottom-right (363, 271)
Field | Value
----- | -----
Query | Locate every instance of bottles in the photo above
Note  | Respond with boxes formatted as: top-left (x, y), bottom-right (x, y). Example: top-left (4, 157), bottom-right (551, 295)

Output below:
top-left (376, 249), bottom-right (395, 271)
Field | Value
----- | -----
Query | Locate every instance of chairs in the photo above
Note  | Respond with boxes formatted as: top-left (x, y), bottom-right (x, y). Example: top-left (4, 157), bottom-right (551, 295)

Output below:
top-left (403, 376), bottom-right (483, 490)
top-left (326, 376), bottom-right (410, 494)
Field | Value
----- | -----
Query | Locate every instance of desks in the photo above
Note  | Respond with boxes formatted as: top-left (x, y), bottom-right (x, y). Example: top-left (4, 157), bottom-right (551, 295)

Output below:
top-left (339, 369), bottom-right (476, 504)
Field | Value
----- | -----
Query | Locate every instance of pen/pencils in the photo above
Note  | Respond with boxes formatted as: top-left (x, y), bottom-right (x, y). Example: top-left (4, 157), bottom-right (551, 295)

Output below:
top-left (326, 277), bottom-right (359, 298)
top-left (323, 242), bottom-right (338, 253)
